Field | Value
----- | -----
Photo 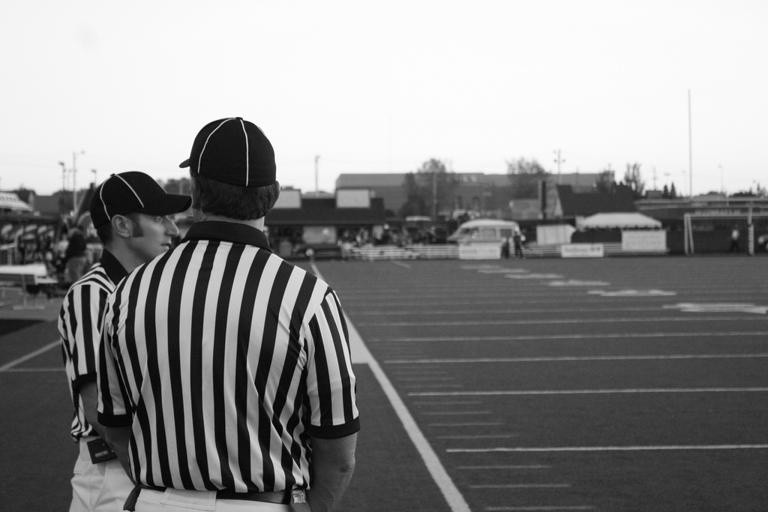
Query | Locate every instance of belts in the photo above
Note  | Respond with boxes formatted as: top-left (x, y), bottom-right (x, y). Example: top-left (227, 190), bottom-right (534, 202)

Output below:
top-left (147, 487), bottom-right (290, 504)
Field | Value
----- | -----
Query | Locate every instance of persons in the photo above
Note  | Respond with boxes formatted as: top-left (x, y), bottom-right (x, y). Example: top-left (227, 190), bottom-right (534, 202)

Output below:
top-left (57, 170), bottom-right (193, 512)
top-left (502, 237), bottom-right (510, 260)
top-left (513, 231), bottom-right (526, 260)
top-left (269, 222), bottom-right (451, 258)
top-left (0, 209), bottom-right (103, 298)
top-left (727, 223), bottom-right (739, 253)
top-left (97, 116), bottom-right (359, 512)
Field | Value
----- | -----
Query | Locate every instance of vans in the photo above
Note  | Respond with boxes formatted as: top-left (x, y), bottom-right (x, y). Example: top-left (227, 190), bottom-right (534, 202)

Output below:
top-left (446, 218), bottom-right (526, 244)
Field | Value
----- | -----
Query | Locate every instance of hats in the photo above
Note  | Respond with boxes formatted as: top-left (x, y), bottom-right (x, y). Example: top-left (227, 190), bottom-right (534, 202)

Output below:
top-left (179, 117), bottom-right (276, 187)
top-left (90, 172), bottom-right (192, 228)
